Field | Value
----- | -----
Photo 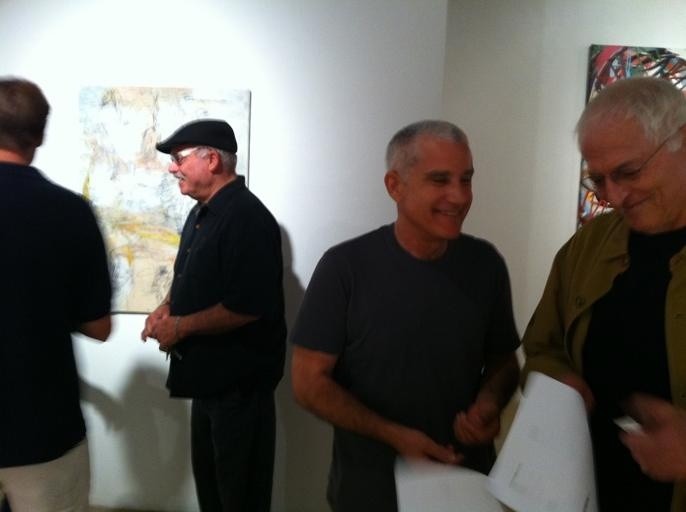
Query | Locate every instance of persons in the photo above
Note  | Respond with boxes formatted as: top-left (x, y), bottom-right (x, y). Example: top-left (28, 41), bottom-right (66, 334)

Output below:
top-left (139, 119), bottom-right (287, 512)
top-left (0, 75), bottom-right (112, 511)
top-left (291, 120), bottom-right (524, 511)
top-left (520, 77), bottom-right (686, 512)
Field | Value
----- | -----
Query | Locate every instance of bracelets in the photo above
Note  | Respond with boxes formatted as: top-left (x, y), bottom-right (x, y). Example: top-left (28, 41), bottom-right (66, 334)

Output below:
top-left (173, 312), bottom-right (184, 345)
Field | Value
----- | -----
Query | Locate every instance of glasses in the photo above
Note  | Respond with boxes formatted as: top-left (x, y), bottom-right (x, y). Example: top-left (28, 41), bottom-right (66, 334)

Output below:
top-left (171, 147), bottom-right (198, 163)
top-left (580, 136), bottom-right (671, 194)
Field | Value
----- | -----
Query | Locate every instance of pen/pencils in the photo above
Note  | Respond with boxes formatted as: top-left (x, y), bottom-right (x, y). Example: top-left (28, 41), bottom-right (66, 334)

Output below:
top-left (170, 348), bottom-right (183, 362)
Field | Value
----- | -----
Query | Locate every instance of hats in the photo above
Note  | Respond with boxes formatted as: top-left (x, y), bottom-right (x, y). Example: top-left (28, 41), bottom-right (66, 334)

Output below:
top-left (155, 118), bottom-right (238, 154)
